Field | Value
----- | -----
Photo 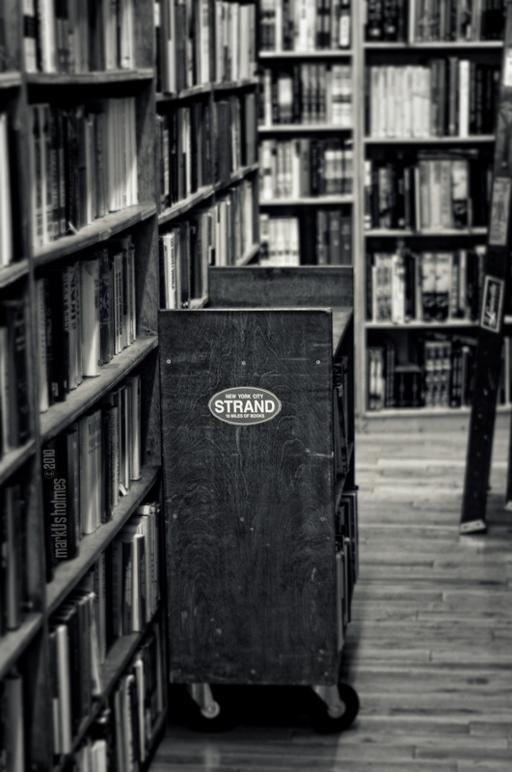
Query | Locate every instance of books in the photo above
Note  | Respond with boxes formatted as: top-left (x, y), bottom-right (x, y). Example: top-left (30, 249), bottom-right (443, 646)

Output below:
top-left (261, 0), bottom-right (512, 412)
top-left (1, 0), bottom-right (269, 770)
